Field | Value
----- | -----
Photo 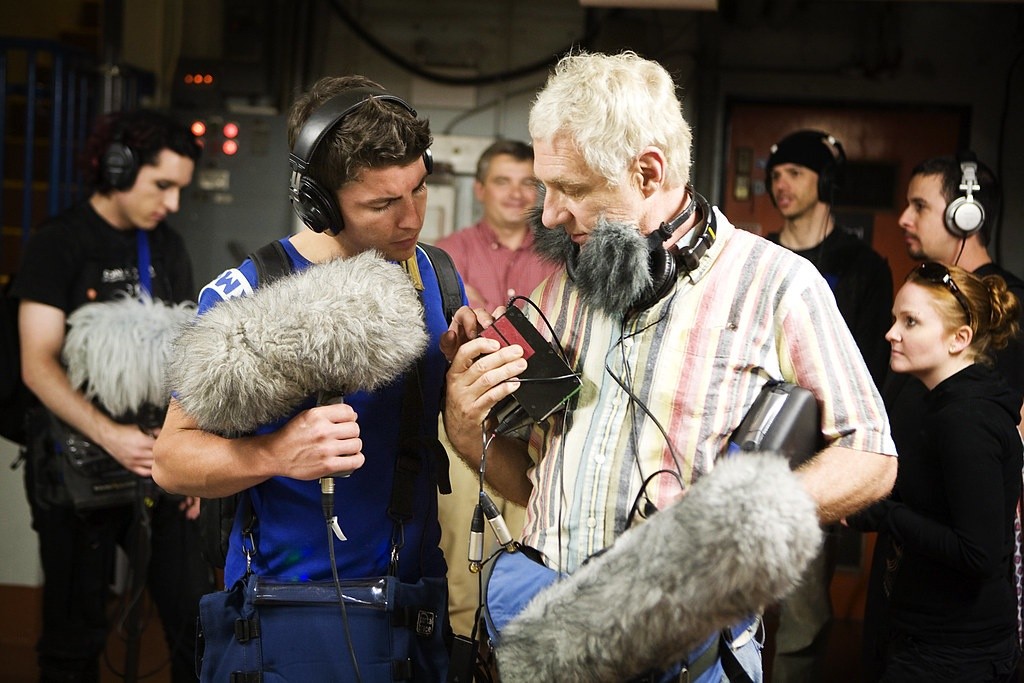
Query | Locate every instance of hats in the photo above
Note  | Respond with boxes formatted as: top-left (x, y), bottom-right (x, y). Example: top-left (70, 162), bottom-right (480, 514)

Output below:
top-left (764, 130), bottom-right (840, 179)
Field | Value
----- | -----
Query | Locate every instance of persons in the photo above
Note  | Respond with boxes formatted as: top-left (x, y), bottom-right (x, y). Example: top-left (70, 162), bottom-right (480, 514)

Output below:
top-left (761, 127), bottom-right (896, 683)
top-left (444, 51), bottom-right (899, 683)
top-left (857, 260), bottom-right (1024, 683)
top-left (150, 75), bottom-right (508, 683)
top-left (898, 151), bottom-right (1024, 421)
top-left (0, 114), bottom-right (217, 683)
top-left (437, 139), bottom-right (563, 641)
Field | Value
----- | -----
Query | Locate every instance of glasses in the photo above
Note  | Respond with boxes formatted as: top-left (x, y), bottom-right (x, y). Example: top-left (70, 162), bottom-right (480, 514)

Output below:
top-left (904, 261), bottom-right (973, 327)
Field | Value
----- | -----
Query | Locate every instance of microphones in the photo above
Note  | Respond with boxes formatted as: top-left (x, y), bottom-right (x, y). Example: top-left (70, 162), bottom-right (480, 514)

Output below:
top-left (494, 449), bottom-right (827, 683)
top-left (165, 248), bottom-right (432, 525)
top-left (60, 282), bottom-right (201, 509)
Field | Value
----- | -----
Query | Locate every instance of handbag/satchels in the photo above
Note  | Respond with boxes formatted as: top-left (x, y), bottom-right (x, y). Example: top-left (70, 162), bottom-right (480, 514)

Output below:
top-left (196, 576), bottom-right (452, 683)
top-left (12, 400), bottom-right (186, 533)
top-left (482, 542), bottom-right (726, 683)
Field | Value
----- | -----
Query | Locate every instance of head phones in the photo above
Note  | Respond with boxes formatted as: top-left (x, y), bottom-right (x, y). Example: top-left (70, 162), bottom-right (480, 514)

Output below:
top-left (288, 86), bottom-right (433, 237)
top-left (943, 151), bottom-right (986, 238)
top-left (566, 182), bottom-right (717, 311)
top-left (816, 136), bottom-right (842, 201)
top-left (103, 108), bottom-right (143, 190)
